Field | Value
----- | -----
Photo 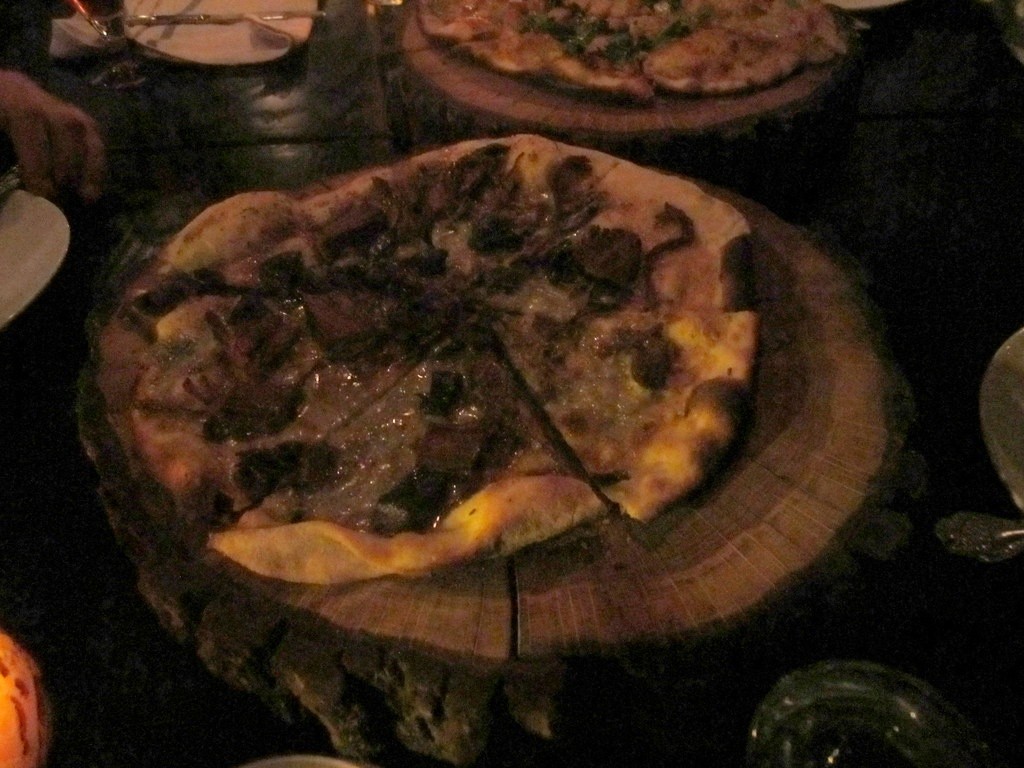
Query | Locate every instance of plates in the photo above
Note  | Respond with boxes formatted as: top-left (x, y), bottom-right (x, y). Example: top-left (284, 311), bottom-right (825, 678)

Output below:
top-left (978, 328), bottom-right (1024, 514)
top-left (0, 190), bottom-right (71, 331)
top-left (121, 0), bottom-right (318, 66)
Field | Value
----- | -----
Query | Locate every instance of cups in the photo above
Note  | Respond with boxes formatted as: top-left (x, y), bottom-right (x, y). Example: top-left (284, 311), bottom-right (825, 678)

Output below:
top-left (745, 659), bottom-right (998, 766)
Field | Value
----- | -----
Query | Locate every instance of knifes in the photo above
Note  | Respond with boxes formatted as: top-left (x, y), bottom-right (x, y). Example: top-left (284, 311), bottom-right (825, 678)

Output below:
top-left (121, 10), bottom-right (326, 28)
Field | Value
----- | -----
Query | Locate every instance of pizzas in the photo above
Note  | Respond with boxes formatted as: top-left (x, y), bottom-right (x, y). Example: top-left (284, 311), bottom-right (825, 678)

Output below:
top-left (129, 132), bottom-right (759, 581)
top-left (410, 1), bottom-right (869, 101)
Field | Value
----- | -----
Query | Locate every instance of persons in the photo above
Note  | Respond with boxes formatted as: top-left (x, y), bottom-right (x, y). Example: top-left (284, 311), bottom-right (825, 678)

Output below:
top-left (0, 71), bottom-right (106, 212)
